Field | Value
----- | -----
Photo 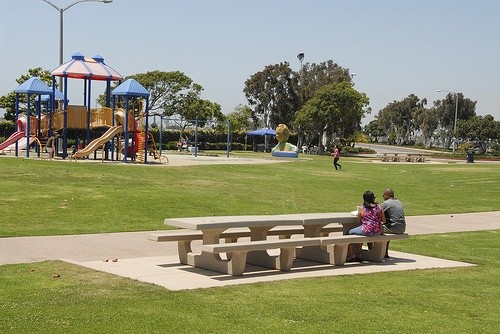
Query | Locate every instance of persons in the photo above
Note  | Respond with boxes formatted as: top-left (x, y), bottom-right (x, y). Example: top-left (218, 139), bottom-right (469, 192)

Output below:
top-left (314, 144), bottom-right (318, 153)
top-left (68, 145), bottom-right (78, 158)
top-left (320, 142), bottom-right (326, 154)
top-left (331, 144), bottom-right (341, 170)
top-left (272, 124), bottom-right (299, 152)
top-left (367, 188), bottom-right (406, 258)
top-left (344, 191), bottom-right (385, 261)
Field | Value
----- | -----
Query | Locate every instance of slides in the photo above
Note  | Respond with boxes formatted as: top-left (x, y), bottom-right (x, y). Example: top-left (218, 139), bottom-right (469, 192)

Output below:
top-left (72, 123), bottom-right (125, 163)
top-left (0, 131), bottom-right (25, 149)
top-left (121, 128), bottom-right (142, 155)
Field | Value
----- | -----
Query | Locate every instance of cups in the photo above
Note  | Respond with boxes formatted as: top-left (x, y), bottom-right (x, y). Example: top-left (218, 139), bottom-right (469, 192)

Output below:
top-left (357, 206), bottom-right (363, 214)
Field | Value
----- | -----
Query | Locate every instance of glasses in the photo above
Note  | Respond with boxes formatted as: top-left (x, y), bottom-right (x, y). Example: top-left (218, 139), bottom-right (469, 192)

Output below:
top-left (374, 197), bottom-right (376, 198)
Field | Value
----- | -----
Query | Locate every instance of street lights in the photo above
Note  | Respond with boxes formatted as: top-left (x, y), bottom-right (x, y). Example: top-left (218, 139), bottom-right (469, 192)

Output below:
top-left (437, 90), bottom-right (458, 155)
top-left (44, 0), bottom-right (114, 111)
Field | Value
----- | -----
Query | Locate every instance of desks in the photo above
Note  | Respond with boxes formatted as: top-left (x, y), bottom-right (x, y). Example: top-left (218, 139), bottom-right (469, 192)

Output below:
top-left (380, 152), bottom-right (434, 163)
top-left (164, 212), bottom-right (359, 275)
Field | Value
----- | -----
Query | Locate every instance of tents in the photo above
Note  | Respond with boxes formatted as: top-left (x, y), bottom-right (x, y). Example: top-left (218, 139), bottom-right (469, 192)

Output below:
top-left (245, 127), bottom-right (276, 153)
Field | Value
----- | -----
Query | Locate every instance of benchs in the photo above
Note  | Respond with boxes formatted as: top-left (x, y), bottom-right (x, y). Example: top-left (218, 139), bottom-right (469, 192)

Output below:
top-left (199, 233), bottom-right (409, 276)
top-left (147, 225), bottom-right (343, 264)
top-left (376, 155), bottom-right (437, 163)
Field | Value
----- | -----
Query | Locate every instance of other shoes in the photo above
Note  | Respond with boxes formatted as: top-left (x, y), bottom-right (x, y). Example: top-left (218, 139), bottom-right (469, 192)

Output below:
top-left (350, 255), bottom-right (359, 262)
top-left (385, 254), bottom-right (389, 258)
